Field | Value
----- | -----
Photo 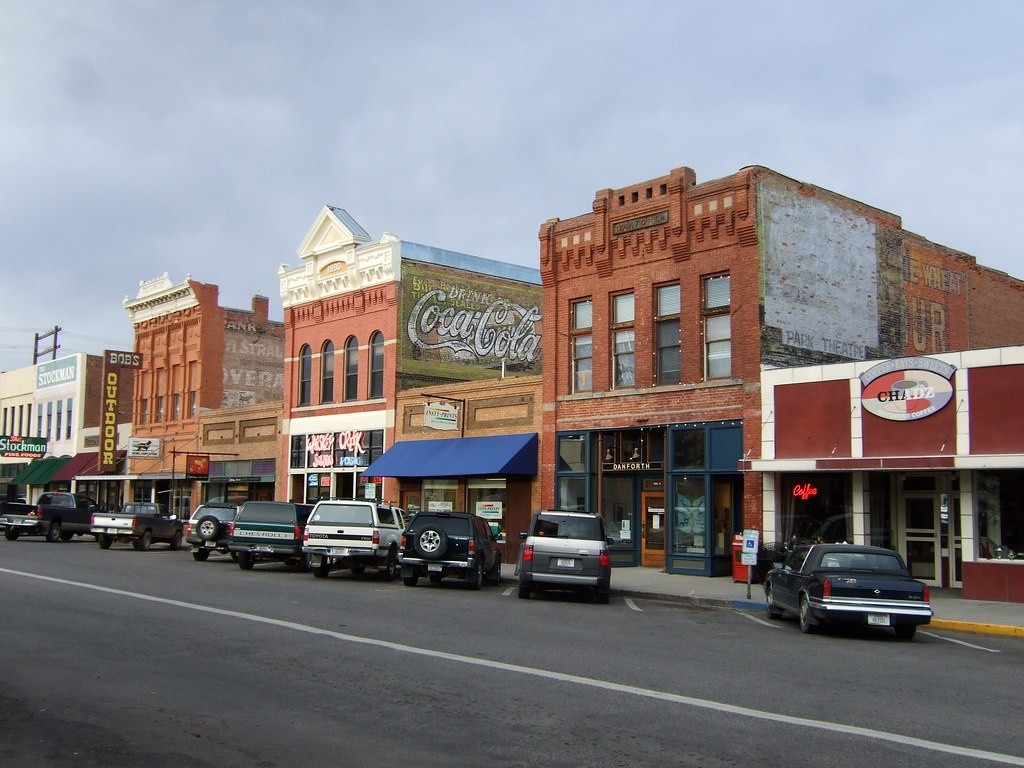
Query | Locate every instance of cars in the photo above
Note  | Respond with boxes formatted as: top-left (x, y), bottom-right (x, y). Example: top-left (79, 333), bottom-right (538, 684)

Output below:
top-left (763, 543), bottom-right (934, 640)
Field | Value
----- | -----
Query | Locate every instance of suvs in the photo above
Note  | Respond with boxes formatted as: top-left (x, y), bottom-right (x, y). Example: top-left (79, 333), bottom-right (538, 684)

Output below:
top-left (186, 502), bottom-right (244, 563)
top-left (302, 496), bottom-right (409, 581)
top-left (227, 500), bottom-right (316, 573)
top-left (398, 511), bottom-right (502, 590)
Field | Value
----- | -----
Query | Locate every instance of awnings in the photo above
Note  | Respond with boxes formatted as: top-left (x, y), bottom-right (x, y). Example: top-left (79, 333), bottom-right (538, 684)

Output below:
top-left (9, 450), bottom-right (127, 484)
top-left (358, 432), bottom-right (538, 476)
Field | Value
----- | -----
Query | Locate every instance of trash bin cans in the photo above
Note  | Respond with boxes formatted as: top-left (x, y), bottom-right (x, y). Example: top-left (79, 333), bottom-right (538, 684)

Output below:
top-left (731, 533), bottom-right (760, 584)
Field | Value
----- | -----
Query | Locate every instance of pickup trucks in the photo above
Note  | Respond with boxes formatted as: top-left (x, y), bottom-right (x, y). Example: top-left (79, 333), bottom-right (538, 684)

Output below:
top-left (0, 491), bottom-right (102, 544)
top-left (89, 501), bottom-right (184, 552)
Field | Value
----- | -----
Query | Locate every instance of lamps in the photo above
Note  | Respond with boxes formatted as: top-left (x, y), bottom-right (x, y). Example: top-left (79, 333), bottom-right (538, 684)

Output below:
top-left (605, 443), bottom-right (613, 460)
top-left (632, 438), bottom-right (640, 458)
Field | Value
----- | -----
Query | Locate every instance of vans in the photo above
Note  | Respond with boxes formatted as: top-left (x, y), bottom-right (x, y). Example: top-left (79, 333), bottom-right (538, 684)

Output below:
top-left (518, 509), bottom-right (614, 605)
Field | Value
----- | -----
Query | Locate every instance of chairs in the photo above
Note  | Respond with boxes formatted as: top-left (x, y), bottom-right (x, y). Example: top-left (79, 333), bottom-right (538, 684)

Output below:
top-left (820, 557), bottom-right (838, 568)
top-left (850, 559), bottom-right (871, 568)
